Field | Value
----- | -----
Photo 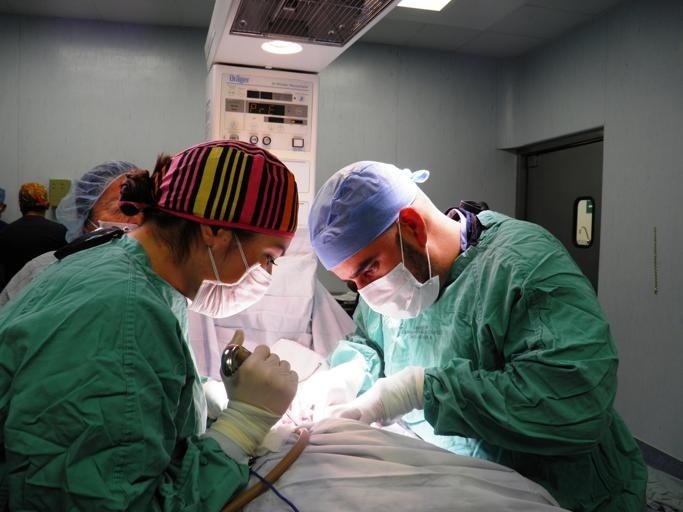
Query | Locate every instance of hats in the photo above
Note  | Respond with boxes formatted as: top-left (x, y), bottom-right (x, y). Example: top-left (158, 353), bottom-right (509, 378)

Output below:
top-left (0, 188), bottom-right (5, 202)
top-left (19, 182), bottom-right (50, 206)
top-left (308, 160), bottom-right (418, 271)
top-left (155, 140), bottom-right (299, 239)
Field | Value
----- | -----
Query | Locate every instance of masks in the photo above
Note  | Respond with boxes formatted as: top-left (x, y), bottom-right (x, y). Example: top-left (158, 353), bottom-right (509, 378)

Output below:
top-left (185, 233), bottom-right (274, 318)
top-left (88, 218), bottom-right (138, 233)
top-left (357, 222), bottom-right (440, 320)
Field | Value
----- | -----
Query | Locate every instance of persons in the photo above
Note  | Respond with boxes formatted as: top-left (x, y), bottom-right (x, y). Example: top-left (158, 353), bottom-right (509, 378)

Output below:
top-left (2, 161), bottom-right (142, 307)
top-left (299, 160), bottom-right (650, 511)
top-left (0, 189), bottom-right (8, 234)
top-left (1, 139), bottom-right (298, 511)
top-left (0, 182), bottom-right (70, 287)
